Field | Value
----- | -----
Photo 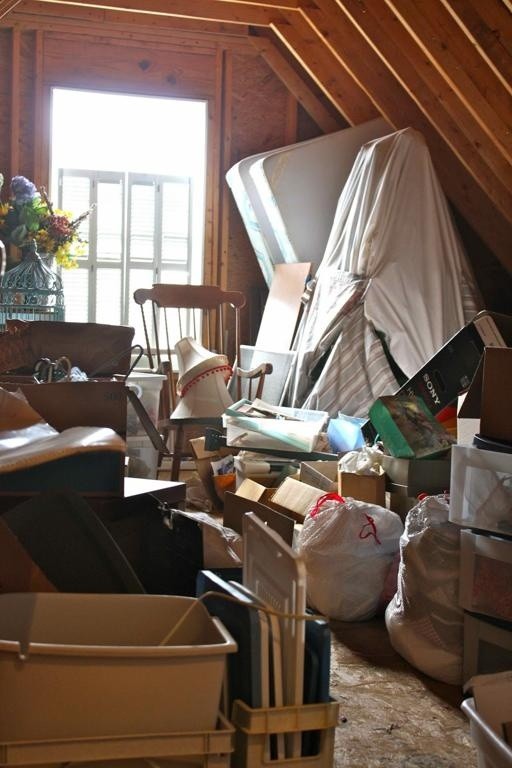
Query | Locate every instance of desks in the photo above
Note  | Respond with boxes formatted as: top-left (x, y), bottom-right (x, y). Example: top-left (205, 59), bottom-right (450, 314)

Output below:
top-left (1, 475), bottom-right (188, 596)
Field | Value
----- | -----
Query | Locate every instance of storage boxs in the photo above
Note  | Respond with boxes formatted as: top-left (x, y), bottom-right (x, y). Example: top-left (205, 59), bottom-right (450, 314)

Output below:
top-left (0, 593), bottom-right (239, 740)
top-left (299, 460), bottom-right (339, 494)
top-left (126, 434), bottom-right (165, 480)
top-left (270, 474), bottom-right (328, 524)
top-left (459, 696), bottom-right (512, 767)
top-left (448, 444), bottom-right (512, 537)
top-left (455, 345), bottom-right (511, 448)
top-left (463, 612), bottom-right (512, 687)
top-left (459, 528), bottom-right (512, 623)
top-left (126, 372), bottom-right (168, 435)
top-left (381, 453), bottom-right (451, 497)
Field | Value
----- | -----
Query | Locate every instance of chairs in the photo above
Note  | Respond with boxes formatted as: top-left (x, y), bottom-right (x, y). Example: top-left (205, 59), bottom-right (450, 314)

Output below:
top-left (134, 282), bottom-right (273, 481)
